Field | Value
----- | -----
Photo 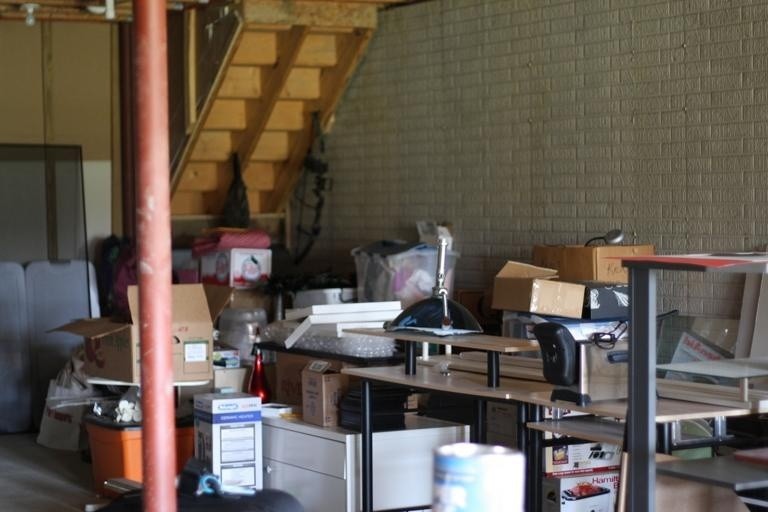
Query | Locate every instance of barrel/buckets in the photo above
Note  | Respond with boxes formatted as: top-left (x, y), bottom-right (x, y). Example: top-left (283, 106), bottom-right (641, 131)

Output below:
top-left (433, 443), bottom-right (527, 512)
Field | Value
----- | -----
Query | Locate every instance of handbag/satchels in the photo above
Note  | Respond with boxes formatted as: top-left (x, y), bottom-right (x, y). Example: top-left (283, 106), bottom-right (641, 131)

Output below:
top-left (36, 367), bottom-right (94, 451)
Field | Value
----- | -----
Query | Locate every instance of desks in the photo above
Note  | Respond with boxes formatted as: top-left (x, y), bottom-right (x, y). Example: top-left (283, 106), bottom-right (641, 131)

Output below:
top-left (340, 326), bottom-right (768, 511)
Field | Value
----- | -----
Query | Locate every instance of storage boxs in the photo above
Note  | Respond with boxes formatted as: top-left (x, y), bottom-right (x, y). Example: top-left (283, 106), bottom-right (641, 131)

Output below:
top-left (206, 248), bottom-right (283, 320)
top-left (78, 411), bottom-right (194, 495)
top-left (193, 389), bottom-right (267, 492)
top-left (489, 241), bottom-right (654, 321)
top-left (67, 282), bottom-right (236, 382)
top-left (298, 358), bottom-right (341, 429)
top-left (483, 402), bottom-right (625, 511)
